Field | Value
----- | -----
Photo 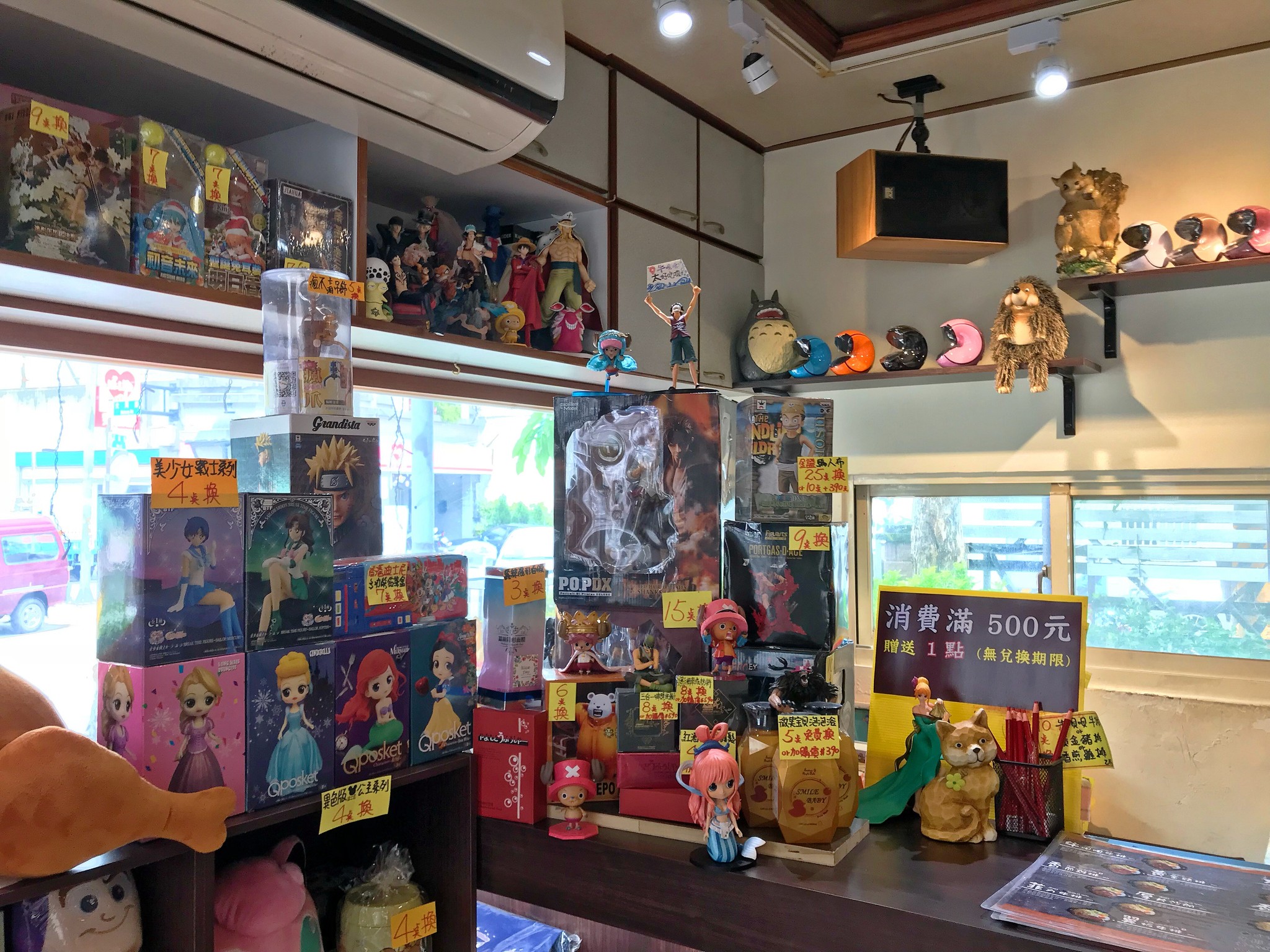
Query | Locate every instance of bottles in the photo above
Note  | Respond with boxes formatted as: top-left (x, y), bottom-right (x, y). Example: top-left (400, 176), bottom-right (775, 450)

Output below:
top-left (737, 701), bottom-right (859, 845)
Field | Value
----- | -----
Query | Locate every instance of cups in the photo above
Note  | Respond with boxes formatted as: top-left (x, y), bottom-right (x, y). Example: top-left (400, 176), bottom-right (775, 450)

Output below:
top-left (338, 882), bottom-right (426, 952)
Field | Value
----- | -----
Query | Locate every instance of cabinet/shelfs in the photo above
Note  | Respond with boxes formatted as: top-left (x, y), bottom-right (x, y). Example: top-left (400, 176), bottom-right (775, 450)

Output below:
top-left (0, 753), bottom-right (475, 952)
top-left (732, 254), bottom-right (1270, 436)
top-left (0, 0), bottom-right (768, 403)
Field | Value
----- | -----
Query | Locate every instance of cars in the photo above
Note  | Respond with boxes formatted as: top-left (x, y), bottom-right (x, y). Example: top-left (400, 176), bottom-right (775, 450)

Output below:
top-left (434, 521), bottom-right (554, 576)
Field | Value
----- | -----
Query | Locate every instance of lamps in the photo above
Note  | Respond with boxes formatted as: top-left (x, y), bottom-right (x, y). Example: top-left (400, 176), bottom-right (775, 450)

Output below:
top-left (1035, 44), bottom-right (1072, 98)
top-left (652, 0), bottom-right (693, 38)
top-left (741, 36), bottom-right (778, 95)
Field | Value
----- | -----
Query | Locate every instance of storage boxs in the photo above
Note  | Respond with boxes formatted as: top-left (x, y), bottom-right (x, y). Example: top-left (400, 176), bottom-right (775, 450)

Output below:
top-left (473, 706), bottom-right (548, 825)
top-left (95, 414), bottom-right (477, 819)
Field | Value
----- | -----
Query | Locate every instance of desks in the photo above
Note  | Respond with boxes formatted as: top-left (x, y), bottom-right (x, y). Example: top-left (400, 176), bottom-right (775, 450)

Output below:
top-left (473, 807), bottom-right (1270, 952)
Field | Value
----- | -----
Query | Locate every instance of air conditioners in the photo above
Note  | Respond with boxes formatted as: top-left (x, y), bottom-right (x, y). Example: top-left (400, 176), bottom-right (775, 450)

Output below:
top-left (0, 0), bottom-right (566, 175)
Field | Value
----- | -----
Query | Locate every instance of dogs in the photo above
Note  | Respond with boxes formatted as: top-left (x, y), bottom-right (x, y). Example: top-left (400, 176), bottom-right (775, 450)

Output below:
top-left (915, 707), bottom-right (1002, 843)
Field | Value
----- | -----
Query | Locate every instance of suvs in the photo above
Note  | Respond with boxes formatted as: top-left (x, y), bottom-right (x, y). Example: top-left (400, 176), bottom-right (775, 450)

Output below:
top-left (0, 515), bottom-right (71, 635)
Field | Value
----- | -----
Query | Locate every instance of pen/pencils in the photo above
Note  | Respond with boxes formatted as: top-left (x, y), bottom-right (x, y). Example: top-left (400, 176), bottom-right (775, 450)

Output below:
top-left (986, 700), bottom-right (1075, 839)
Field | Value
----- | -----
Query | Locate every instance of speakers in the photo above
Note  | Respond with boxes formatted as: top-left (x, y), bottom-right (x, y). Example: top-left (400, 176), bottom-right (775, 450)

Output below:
top-left (834, 149), bottom-right (1009, 265)
